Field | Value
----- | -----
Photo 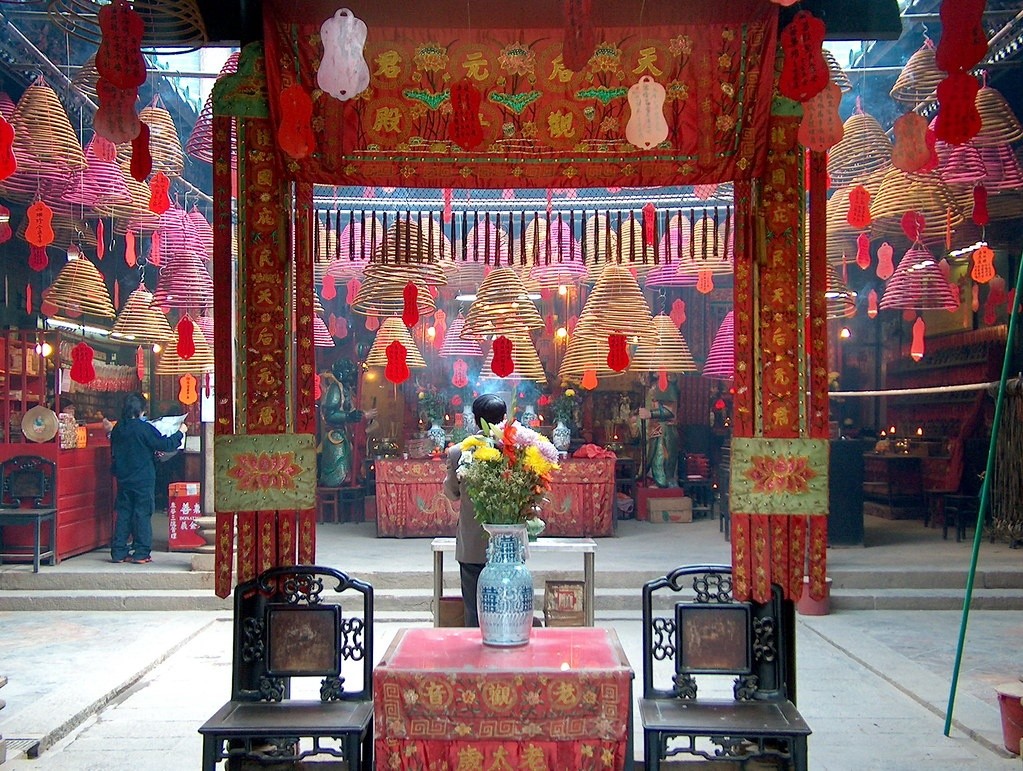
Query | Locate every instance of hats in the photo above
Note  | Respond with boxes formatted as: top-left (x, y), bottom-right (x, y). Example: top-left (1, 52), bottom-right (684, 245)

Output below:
top-left (471, 393), bottom-right (506, 423)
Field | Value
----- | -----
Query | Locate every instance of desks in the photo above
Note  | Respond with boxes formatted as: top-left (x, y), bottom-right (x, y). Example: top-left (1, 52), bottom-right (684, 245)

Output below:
top-left (865, 453), bottom-right (925, 521)
top-left (430, 537), bottom-right (597, 627)
top-left (371, 627), bottom-right (634, 771)
top-left (616, 455), bottom-right (636, 519)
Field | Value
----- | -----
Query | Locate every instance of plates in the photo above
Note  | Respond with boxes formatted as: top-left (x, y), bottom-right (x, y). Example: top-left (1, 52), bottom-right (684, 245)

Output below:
top-left (21, 406), bottom-right (59, 442)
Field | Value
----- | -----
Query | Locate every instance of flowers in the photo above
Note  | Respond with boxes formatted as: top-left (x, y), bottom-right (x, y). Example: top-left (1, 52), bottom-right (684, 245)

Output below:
top-left (412, 377), bottom-right (583, 435)
top-left (455, 391), bottom-right (561, 539)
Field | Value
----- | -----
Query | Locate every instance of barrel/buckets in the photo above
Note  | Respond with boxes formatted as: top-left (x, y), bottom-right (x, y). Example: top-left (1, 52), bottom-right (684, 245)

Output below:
top-left (545, 580), bottom-right (585, 627)
top-left (439, 597), bottom-right (464, 627)
top-left (996, 682), bottom-right (1023, 756)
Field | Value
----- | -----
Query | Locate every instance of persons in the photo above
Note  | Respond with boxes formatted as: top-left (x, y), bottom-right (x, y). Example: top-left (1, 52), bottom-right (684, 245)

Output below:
top-left (635, 372), bottom-right (678, 488)
top-left (60, 400), bottom-right (83, 429)
top-left (443, 395), bottom-right (506, 627)
top-left (320, 358), bottom-right (378, 487)
top-left (103, 392), bottom-right (188, 564)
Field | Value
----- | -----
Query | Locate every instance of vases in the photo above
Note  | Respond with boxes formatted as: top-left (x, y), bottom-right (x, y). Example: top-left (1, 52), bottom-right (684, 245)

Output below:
top-left (553, 418), bottom-right (570, 451)
top-left (476, 523), bottom-right (533, 647)
top-left (526, 517), bottom-right (546, 541)
top-left (461, 406), bottom-right (475, 431)
top-left (521, 405), bottom-right (538, 428)
top-left (428, 419), bottom-right (446, 452)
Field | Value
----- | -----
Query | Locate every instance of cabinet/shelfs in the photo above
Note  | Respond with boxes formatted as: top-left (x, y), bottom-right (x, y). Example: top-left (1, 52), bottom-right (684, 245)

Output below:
top-left (0, 336), bottom-right (47, 443)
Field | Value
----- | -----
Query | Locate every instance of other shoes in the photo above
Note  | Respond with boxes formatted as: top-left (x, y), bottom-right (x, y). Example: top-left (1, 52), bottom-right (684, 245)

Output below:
top-left (111, 554), bottom-right (131, 564)
top-left (130, 555), bottom-right (152, 564)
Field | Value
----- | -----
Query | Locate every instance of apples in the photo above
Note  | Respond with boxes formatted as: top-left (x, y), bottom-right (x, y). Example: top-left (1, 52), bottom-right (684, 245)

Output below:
top-left (431, 446), bottom-right (441, 454)
top-left (94, 410), bottom-right (103, 418)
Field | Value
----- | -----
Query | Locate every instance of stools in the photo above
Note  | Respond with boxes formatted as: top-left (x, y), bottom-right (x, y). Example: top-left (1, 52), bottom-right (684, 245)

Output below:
top-left (320, 487), bottom-right (365, 525)
top-left (942, 495), bottom-right (981, 542)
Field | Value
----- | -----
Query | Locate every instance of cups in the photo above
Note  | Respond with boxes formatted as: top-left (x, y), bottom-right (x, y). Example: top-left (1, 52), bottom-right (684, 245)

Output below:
top-left (403, 452), bottom-right (408, 459)
top-left (562, 452), bottom-right (568, 458)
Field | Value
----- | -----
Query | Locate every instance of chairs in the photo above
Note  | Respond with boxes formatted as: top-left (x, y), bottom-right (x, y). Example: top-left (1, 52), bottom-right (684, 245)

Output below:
top-left (198, 565), bottom-right (374, 771)
top-left (638, 565), bottom-right (812, 771)
top-left (0, 455), bottom-right (57, 572)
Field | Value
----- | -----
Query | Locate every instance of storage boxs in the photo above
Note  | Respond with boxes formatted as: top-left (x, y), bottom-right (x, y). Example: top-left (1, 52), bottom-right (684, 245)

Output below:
top-left (543, 580), bottom-right (584, 628)
top-left (439, 598), bottom-right (465, 627)
top-left (647, 497), bottom-right (692, 523)
